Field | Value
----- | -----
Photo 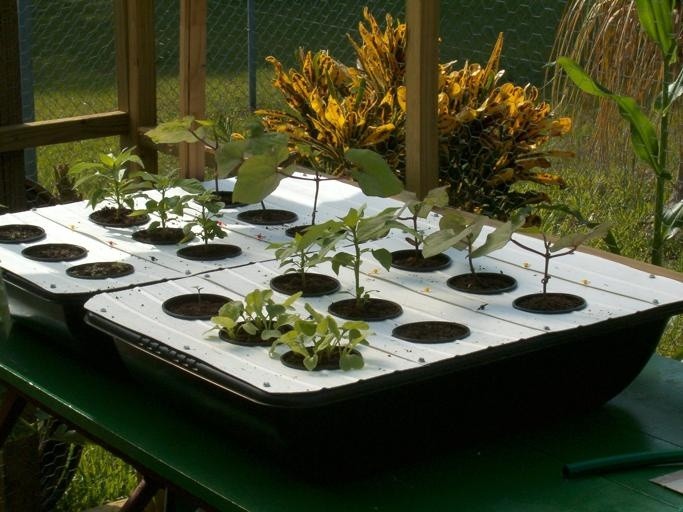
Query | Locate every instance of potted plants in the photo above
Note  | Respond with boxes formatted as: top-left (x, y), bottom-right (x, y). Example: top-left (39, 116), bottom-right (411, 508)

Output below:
top-left (0, 116), bottom-right (415, 343)
top-left (81, 114), bottom-right (683, 478)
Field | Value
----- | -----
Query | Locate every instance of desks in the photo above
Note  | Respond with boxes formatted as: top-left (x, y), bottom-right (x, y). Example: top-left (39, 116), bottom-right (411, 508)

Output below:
top-left (0, 322), bottom-right (682, 512)
